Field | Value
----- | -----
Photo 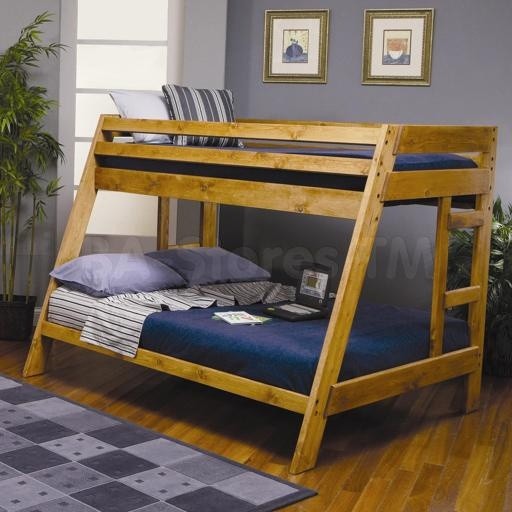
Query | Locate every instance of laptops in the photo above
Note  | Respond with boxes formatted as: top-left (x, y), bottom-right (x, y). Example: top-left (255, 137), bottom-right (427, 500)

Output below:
top-left (265, 261), bottom-right (334, 323)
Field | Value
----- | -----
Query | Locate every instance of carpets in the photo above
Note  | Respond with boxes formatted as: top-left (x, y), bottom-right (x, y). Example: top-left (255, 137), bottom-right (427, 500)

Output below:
top-left (0, 369), bottom-right (320, 510)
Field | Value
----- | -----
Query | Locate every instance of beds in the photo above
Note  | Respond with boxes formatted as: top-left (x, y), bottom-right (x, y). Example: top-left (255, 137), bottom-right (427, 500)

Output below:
top-left (26, 119), bottom-right (499, 477)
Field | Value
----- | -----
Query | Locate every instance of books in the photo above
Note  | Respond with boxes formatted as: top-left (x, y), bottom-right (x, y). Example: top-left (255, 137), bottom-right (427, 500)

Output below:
top-left (213, 306), bottom-right (264, 327)
top-left (211, 314), bottom-right (274, 322)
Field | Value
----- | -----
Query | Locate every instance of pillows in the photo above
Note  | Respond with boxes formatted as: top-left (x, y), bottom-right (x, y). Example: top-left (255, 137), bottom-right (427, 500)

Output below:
top-left (108, 83), bottom-right (251, 150)
top-left (47, 243), bottom-right (273, 299)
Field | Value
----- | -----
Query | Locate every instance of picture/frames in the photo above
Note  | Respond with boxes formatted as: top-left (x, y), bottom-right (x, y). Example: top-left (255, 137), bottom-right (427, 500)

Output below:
top-left (260, 7), bottom-right (331, 86)
top-left (361, 8), bottom-right (435, 89)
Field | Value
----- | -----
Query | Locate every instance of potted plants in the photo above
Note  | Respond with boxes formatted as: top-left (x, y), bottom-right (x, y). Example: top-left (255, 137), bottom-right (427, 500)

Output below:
top-left (0, 17), bottom-right (69, 342)
top-left (431, 196), bottom-right (511, 382)
